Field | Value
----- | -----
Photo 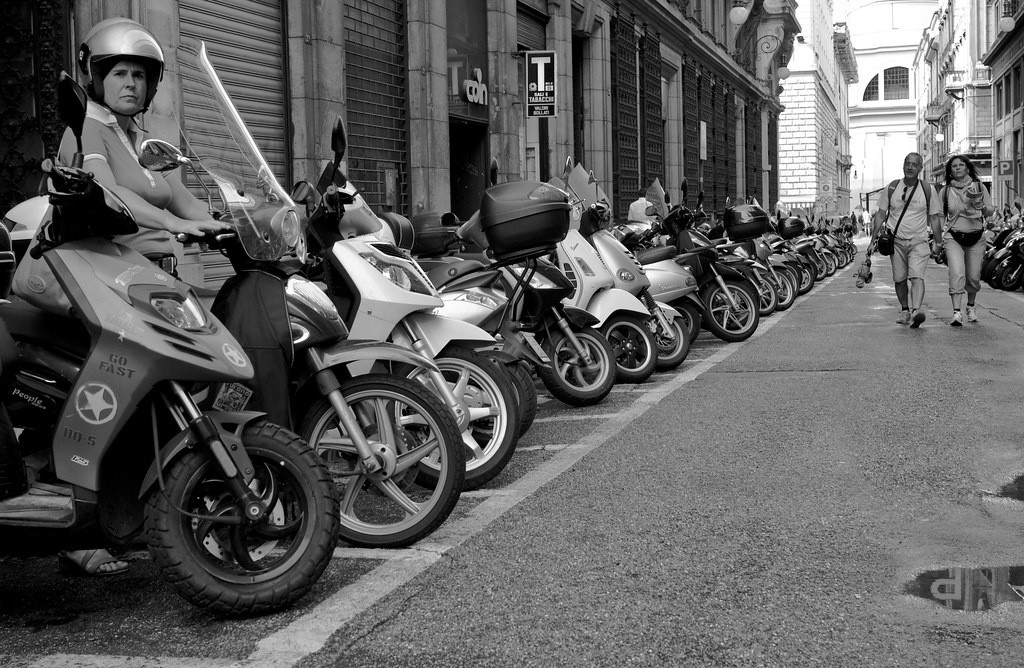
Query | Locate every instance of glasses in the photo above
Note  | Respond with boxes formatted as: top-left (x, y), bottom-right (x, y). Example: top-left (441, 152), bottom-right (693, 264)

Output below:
top-left (902, 186), bottom-right (908, 202)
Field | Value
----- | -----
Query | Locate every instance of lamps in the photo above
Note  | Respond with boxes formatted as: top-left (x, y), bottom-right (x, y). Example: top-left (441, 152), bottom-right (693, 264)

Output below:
top-left (838, 156), bottom-right (851, 176)
top-left (729, 0), bottom-right (749, 25)
top-left (821, 129), bottom-right (839, 152)
top-left (936, 114), bottom-right (951, 141)
top-left (1000, 0), bottom-right (1015, 33)
top-left (753, 35), bottom-right (790, 79)
top-left (852, 164), bottom-right (858, 179)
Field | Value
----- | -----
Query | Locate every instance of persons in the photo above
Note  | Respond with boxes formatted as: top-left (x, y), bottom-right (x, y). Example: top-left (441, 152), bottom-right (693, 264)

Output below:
top-left (937, 156), bottom-right (995, 327)
top-left (627, 188), bottom-right (658, 225)
top-left (838, 207), bottom-right (877, 236)
top-left (56, 16), bottom-right (232, 576)
top-left (867, 153), bottom-right (943, 329)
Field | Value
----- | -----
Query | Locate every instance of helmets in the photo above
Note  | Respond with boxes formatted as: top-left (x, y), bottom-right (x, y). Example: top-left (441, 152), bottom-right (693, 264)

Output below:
top-left (78, 16), bottom-right (165, 106)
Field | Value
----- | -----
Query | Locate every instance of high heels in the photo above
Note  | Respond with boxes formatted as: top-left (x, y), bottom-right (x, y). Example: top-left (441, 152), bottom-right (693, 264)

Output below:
top-left (58, 549), bottom-right (131, 574)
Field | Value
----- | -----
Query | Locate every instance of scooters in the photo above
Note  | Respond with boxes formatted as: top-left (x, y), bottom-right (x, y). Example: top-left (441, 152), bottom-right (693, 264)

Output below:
top-left (290, 109), bottom-right (1024, 495)
top-left (0, 69), bottom-right (341, 622)
top-left (0, 37), bottom-right (465, 549)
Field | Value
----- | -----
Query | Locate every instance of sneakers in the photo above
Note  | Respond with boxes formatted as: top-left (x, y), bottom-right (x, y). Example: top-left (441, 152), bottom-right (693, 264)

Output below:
top-left (896, 311), bottom-right (911, 324)
top-left (966, 305), bottom-right (979, 323)
top-left (909, 310), bottom-right (925, 328)
top-left (950, 311), bottom-right (963, 326)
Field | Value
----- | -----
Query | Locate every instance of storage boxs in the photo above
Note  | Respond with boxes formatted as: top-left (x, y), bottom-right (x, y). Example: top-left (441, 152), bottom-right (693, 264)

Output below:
top-left (377, 180), bottom-right (805, 254)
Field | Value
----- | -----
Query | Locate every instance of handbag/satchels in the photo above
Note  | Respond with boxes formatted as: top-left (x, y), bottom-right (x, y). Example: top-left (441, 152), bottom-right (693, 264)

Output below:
top-left (878, 233), bottom-right (895, 256)
top-left (948, 228), bottom-right (985, 247)
